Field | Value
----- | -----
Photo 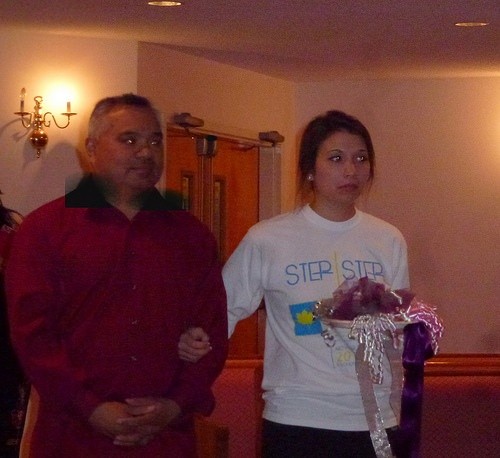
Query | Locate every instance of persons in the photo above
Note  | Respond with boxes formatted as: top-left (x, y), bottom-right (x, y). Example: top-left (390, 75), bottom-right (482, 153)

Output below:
top-left (4, 93), bottom-right (229, 458)
top-left (176, 110), bottom-right (411, 458)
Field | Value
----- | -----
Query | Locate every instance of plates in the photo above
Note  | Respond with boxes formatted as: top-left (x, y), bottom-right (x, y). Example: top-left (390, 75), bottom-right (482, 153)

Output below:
top-left (326, 319), bottom-right (405, 331)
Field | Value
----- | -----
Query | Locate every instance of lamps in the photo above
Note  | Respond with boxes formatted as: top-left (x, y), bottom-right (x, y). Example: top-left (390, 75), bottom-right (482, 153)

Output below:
top-left (14, 86), bottom-right (77, 159)
